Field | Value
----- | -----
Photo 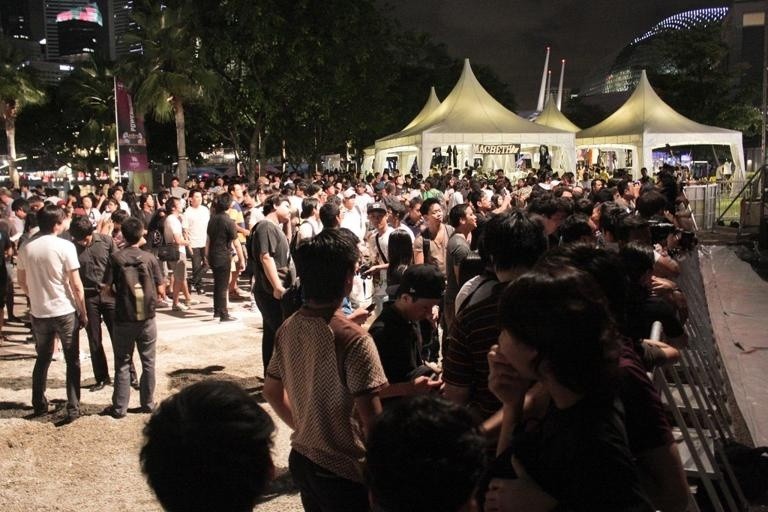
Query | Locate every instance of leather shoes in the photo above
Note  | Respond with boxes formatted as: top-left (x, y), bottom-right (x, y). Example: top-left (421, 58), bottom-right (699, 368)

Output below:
top-left (35, 379), bottom-right (154, 424)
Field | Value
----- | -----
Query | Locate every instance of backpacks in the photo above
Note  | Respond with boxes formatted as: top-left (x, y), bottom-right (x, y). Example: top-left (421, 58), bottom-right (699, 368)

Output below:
top-left (117, 250), bottom-right (156, 324)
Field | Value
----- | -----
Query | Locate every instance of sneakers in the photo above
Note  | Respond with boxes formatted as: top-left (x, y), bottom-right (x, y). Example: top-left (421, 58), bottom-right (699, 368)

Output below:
top-left (156, 277), bottom-right (252, 321)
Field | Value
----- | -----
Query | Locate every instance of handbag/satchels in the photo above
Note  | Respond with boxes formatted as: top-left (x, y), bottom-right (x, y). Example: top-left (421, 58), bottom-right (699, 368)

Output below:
top-left (157, 245), bottom-right (180, 262)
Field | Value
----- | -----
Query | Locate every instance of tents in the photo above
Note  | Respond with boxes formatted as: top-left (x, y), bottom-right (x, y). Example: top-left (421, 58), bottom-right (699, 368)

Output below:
top-left (366, 59), bottom-right (744, 182)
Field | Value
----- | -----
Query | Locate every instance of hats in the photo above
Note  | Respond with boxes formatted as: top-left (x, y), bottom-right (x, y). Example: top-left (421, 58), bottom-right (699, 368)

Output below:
top-left (367, 202), bottom-right (388, 214)
top-left (344, 190), bottom-right (357, 198)
top-left (385, 264), bottom-right (446, 300)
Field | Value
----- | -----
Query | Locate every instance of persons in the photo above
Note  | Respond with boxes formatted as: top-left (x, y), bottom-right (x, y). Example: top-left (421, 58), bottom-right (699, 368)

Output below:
top-left (0, 147), bottom-right (731, 512)
